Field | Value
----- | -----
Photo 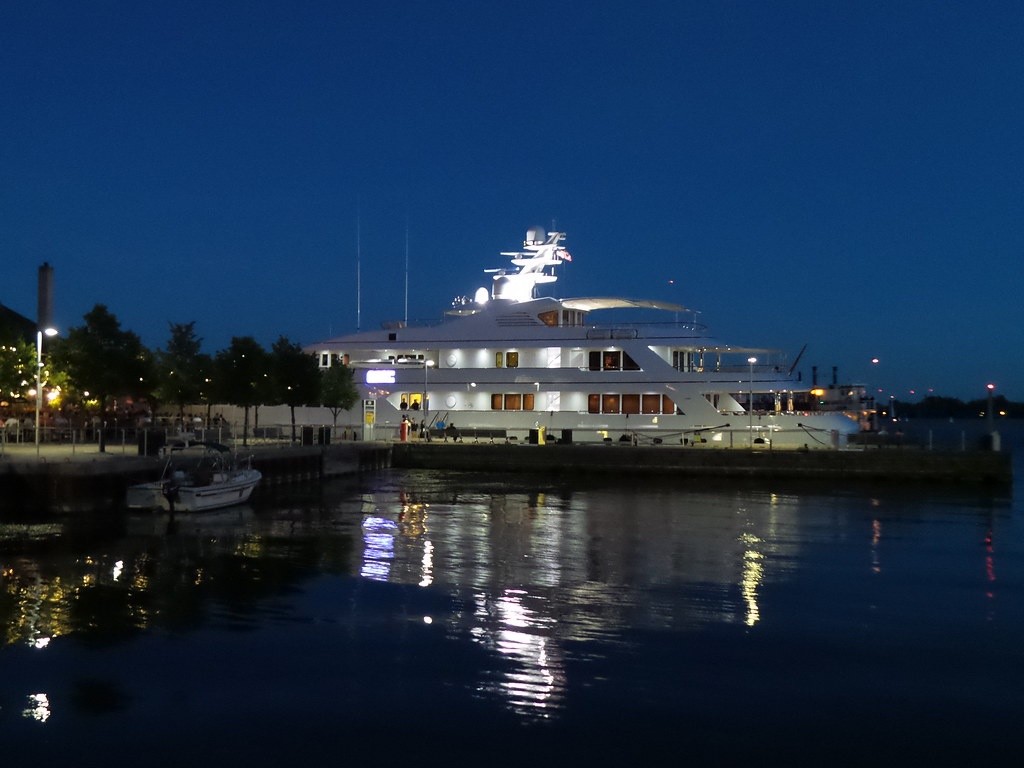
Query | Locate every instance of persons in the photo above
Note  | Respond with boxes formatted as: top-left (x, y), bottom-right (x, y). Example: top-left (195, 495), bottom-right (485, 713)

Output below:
top-left (774, 366), bottom-right (779, 373)
top-left (402, 414), bottom-right (457, 442)
top-left (878, 425), bottom-right (905, 449)
top-left (0, 404), bottom-right (227, 443)
top-left (400, 398), bottom-right (419, 411)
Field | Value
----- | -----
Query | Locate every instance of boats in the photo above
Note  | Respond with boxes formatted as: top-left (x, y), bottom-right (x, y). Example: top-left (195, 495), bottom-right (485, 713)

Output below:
top-left (298, 218), bottom-right (863, 451)
top-left (125, 441), bottom-right (263, 514)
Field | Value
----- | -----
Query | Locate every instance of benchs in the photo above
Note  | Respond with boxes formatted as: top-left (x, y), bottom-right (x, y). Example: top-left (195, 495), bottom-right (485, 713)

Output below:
top-left (429, 428), bottom-right (507, 444)
top-left (252, 427), bottom-right (283, 442)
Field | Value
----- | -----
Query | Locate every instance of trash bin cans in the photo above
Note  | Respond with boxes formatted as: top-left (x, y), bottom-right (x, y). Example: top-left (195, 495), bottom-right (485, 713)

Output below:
top-left (562, 429), bottom-right (572, 444)
top-left (302, 426), bottom-right (313, 447)
top-left (529, 429), bottom-right (538, 444)
top-left (138, 428), bottom-right (166, 458)
top-left (318, 426), bottom-right (331, 447)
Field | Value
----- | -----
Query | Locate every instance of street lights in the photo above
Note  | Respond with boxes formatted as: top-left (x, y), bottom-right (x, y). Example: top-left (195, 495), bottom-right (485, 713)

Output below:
top-left (988, 384), bottom-right (994, 436)
top-left (35, 327), bottom-right (58, 457)
top-left (424, 359), bottom-right (435, 442)
top-left (748, 357), bottom-right (757, 448)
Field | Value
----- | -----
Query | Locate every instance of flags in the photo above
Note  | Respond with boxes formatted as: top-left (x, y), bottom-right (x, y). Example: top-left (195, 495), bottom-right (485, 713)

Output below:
top-left (555, 248), bottom-right (572, 262)
